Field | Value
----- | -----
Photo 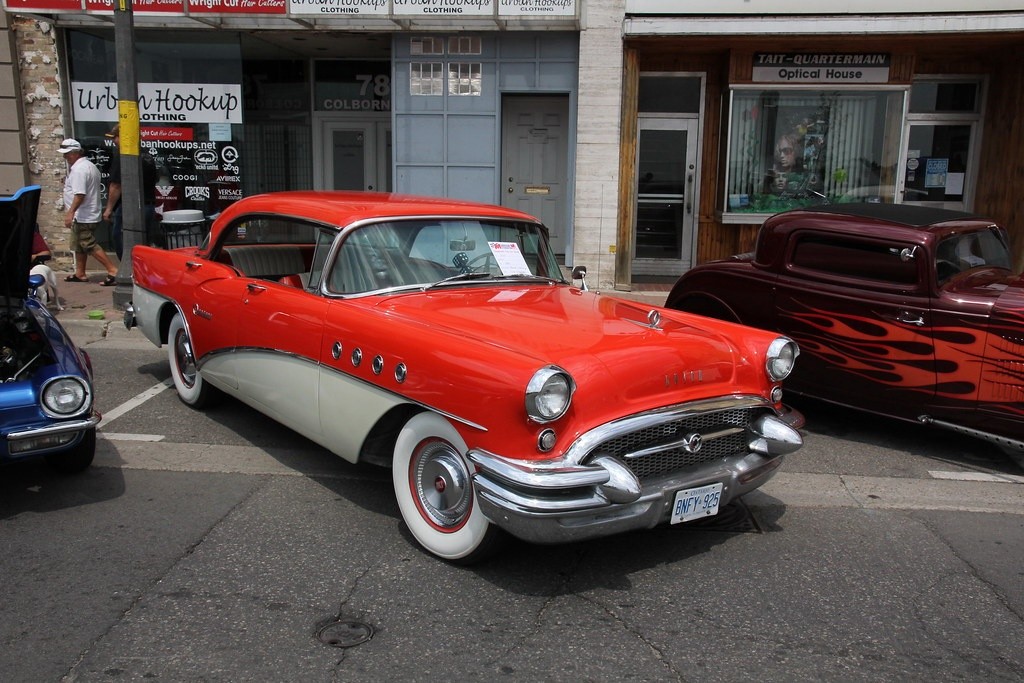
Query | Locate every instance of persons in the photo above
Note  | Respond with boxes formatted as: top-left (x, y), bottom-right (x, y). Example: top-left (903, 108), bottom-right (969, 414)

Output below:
top-left (57, 138), bottom-right (119, 286)
top-left (769, 131), bottom-right (802, 196)
top-left (103, 123), bottom-right (168, 262)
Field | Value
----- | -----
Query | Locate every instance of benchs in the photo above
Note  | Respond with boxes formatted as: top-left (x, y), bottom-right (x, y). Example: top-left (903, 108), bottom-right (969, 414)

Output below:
top-left (224, 245), bottom-right (307, 282)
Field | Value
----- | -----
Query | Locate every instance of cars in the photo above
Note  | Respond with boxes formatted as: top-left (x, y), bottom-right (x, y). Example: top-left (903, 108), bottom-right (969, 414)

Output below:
top-left (661, 205), bottom-right (1024, 455)
top-left (126, 188), bottom-right (809, 565)
top-left (0, 184), bottom-right (103, 477)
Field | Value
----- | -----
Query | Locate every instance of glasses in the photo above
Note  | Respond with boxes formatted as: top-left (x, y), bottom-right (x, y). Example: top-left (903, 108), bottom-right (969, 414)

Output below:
top-left (60, 144), bottom-right (79, 148)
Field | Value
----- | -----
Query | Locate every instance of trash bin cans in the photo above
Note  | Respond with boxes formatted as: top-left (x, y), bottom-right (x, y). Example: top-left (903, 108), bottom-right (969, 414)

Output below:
top-left (159, 210), bottom-right (206, 250)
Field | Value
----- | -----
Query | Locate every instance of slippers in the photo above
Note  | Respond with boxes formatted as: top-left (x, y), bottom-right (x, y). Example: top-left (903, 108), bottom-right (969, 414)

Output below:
top-left (100, 275), bottom-right (116, 285)
top-left (64, 274), bottom-right (89, 282)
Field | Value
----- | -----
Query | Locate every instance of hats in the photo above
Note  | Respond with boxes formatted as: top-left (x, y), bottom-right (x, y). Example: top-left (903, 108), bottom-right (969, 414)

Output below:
top-left (105, 124), bottom-right (119, 137)
top-left (56, 138), bottom-right (82, 153)
